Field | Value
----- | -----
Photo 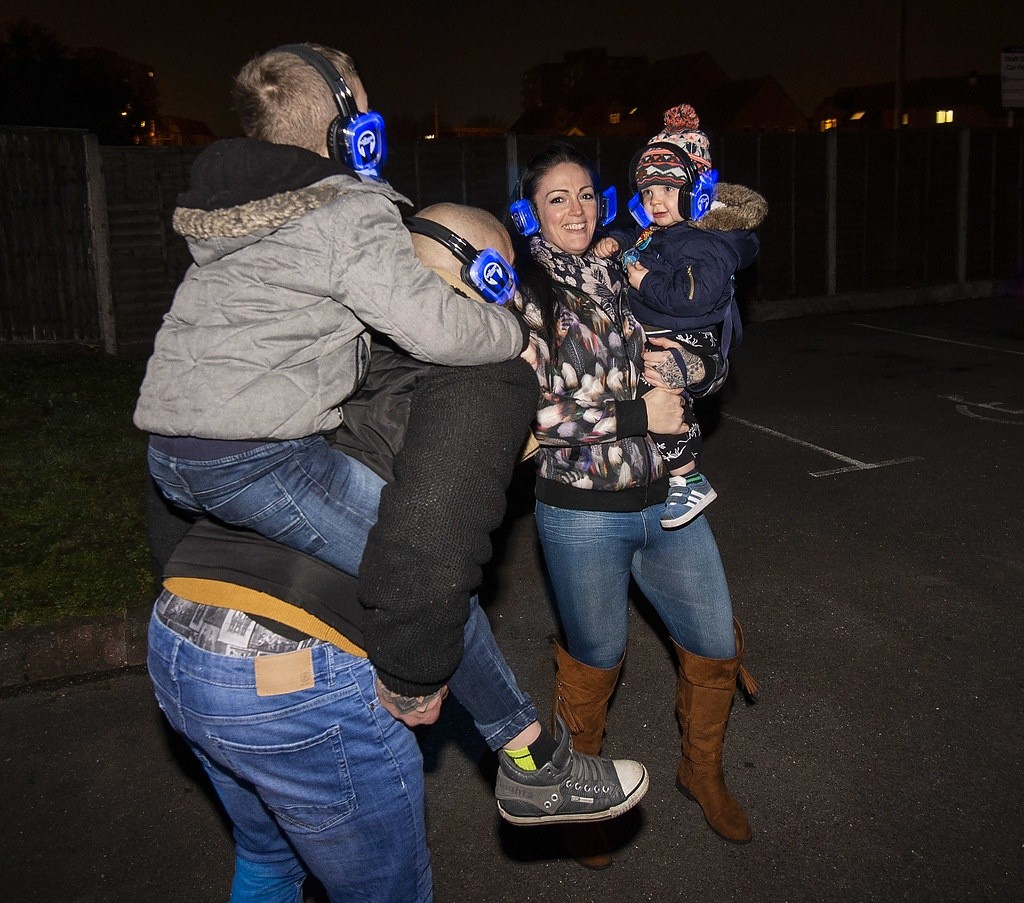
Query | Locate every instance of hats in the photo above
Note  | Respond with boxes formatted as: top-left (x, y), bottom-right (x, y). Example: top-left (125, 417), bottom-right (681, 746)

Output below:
top-left (635, 105), bottom-right (712, 190)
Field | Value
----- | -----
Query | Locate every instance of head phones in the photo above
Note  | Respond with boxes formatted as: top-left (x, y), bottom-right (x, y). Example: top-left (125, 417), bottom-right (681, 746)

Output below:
top-left (627, 141), bottom-right (713, 229)
top-left (401, 215), bottom-right (518, 305)
top-left (272, 45), bottom-right (387, 179)
top-left (508, 166), bottom-right (617, 235)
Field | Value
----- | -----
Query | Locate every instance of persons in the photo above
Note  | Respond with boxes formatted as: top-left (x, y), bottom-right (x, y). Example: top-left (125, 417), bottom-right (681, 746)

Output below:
top-left (593, 129), bottom-right (769, 528)
top-left (503, 136), bottom-right (756, 873)
top-left (146, 199), bottom-right (538, 903)
top-left (132, 42), bottom-right (649, 825)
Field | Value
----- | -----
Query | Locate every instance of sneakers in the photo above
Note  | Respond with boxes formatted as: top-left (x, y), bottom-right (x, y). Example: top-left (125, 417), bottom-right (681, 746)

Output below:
top-left (659, 473), bottom-right (717, 528)
top-left (494, 713), bottom-right (649, 827)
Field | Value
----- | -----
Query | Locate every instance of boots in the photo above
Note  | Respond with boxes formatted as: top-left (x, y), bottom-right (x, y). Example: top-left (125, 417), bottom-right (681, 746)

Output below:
top-left (553, 636), bottom-right (626, 869)
top-left (670, 616), bottom-right (759, 844)
